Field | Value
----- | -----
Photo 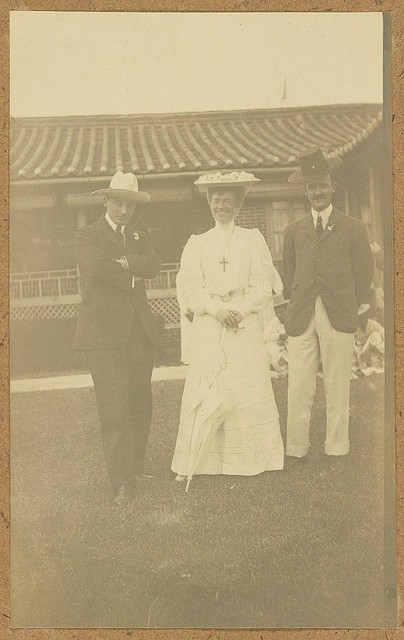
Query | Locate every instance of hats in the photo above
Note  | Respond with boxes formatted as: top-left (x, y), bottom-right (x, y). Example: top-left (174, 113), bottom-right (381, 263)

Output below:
top-left (286, 147), bottom-right (343, 184)
top-left (91, 171), bottom-right (152, 204)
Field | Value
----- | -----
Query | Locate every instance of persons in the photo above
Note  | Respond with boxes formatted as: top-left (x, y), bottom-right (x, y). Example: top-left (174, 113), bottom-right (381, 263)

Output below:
top-left (72, 170), bottom-right (162, 508)
top-left (169, 172), bottom-right (286, 479)
top-left (282, 146), bottom-right (376, 473)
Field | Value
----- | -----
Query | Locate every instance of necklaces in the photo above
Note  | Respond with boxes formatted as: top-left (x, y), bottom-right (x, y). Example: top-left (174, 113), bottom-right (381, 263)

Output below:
top-left (214, 224), bottom-right (236, 273)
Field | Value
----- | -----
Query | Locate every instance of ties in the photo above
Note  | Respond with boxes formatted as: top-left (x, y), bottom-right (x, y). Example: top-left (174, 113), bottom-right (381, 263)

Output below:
top-left (316, 217), bottom-right (324, 236)
top-left (116, 226), bottom-right (125, 242)
